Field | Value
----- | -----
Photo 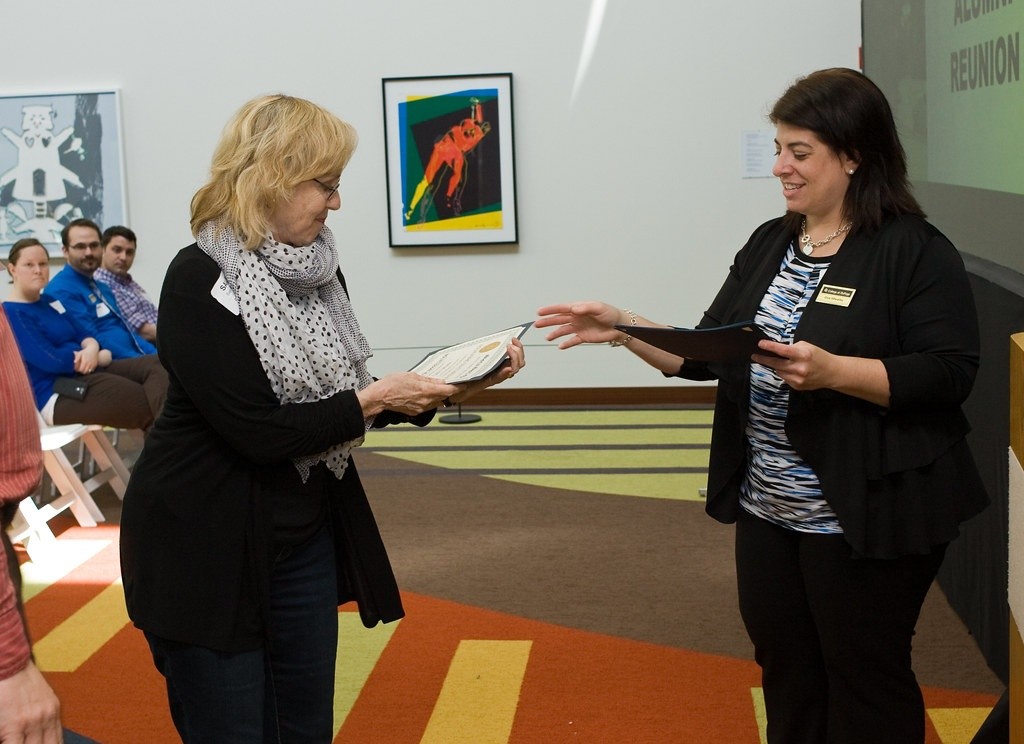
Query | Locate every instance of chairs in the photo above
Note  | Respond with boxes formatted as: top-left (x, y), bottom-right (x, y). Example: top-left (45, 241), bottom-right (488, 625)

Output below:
top-left (2, 401), bottom-right (149, 574)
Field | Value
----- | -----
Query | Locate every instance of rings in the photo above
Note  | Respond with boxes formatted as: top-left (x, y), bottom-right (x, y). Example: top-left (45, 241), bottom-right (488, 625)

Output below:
top-left (88, 368), bottom-right (93, 374)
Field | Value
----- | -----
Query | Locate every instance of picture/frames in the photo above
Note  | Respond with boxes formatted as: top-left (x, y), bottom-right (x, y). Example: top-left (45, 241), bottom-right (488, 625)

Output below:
top-left (0, 87), bottom-right (131, 264)
top-left (381, 72), bottom-right (520, 248)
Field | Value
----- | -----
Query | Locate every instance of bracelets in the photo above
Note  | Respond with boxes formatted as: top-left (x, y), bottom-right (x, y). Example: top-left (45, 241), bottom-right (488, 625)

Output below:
top-left (606, 307), bottom-right (638, 348)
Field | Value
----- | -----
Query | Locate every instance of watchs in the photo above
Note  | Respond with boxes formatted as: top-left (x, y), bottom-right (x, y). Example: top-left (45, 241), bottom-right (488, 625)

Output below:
top-left (441, 396), bottom-right (456, 408)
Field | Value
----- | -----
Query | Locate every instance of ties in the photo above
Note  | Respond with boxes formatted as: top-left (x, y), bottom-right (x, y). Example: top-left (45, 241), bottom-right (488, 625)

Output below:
top-left (89, 281), bottom-right (147, 355)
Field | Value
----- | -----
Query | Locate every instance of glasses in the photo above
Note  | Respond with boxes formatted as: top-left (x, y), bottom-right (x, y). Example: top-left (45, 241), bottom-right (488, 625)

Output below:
top-left (65, 241), bottom-right (103, 252)
top-left (312, 175), bottom-right (342, 201)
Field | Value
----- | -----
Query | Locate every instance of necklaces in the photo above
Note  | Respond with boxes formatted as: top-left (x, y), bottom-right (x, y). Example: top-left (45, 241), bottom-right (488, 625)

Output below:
top-left (801, 219), bottom-right (855, 257)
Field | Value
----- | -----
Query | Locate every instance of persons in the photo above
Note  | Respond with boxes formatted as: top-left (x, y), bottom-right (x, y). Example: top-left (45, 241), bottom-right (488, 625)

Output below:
top-left (118, 92), bottom-right (527, 744)
top-left (93, 225), bottom-right (158, 348)
top-left (2, 237), bottom-right (171, 440)
top-left (533, 66), bottom-right (993, 744)
top-left (0, 304), bottom-right (65, 744)
top-left (43, 218), bottom-right (158, 360)
